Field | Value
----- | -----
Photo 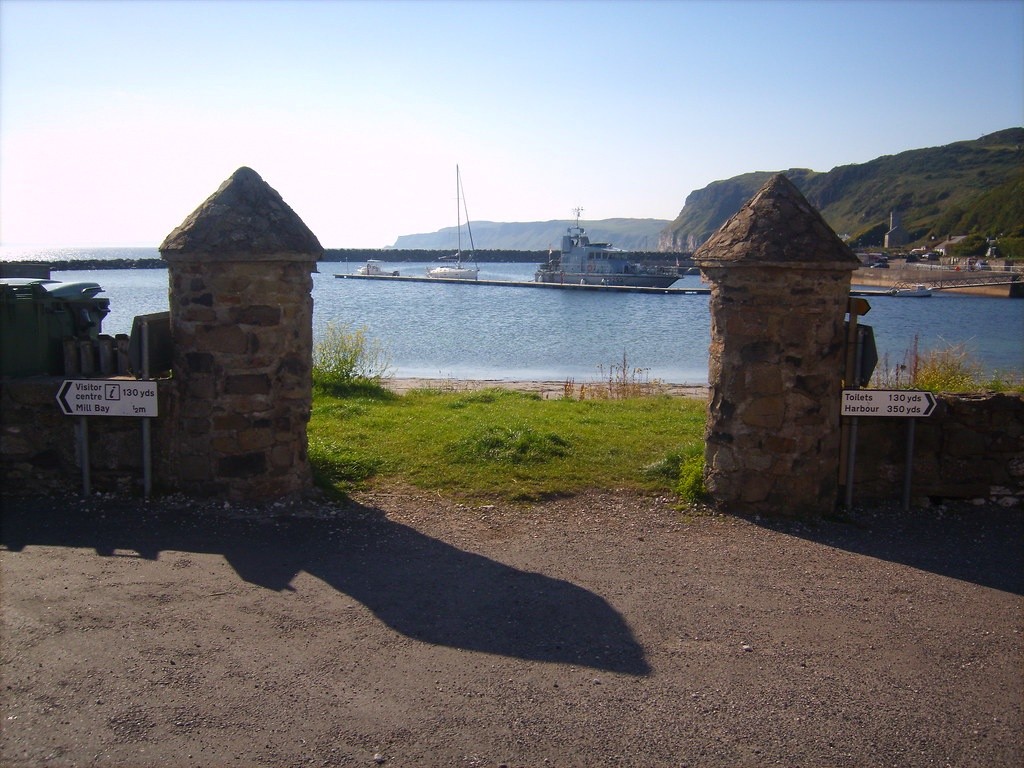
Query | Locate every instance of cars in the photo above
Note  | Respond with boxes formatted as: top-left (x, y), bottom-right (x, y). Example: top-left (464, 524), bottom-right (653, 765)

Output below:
top-left (921, 252), bottom-right (993, 273)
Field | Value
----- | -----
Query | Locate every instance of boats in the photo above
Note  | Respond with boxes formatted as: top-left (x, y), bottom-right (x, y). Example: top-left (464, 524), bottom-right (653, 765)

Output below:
top-left (356, 259), bottom-right (401, 275)
top-left (536, 226), bottom-right (685, 287)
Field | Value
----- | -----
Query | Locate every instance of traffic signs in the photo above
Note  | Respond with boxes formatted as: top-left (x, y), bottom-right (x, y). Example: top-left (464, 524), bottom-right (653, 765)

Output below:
top-left (840, 389), bottom-right (938, 417)
top-left (56, 378), bottom-right (160, 418)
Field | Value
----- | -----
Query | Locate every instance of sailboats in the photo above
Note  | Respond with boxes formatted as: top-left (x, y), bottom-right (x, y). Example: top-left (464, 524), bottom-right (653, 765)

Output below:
top-left (425, 164), bottom-right (480, 280)
